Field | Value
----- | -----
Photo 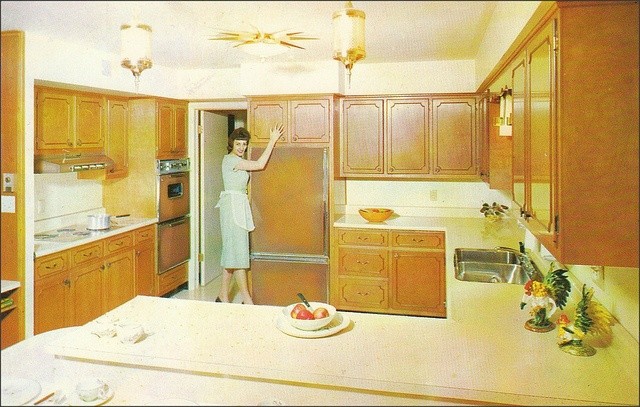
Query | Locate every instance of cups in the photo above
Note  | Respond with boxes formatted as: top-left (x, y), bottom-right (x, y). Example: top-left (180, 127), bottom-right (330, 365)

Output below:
top-left (76, 378), bottom-right (106, 401)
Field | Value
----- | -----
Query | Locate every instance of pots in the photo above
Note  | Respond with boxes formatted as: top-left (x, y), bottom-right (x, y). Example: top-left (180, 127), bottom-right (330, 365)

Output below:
top-left (85, 212), bottom-right (130, 230)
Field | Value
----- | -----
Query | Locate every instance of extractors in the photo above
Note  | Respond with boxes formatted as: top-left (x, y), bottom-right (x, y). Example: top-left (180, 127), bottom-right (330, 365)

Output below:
top-left (35, 155), bottom-right (114, 177)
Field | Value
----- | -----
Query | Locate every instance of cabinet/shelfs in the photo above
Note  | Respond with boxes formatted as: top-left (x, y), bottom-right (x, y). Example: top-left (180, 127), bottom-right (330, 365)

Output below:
top-left (102, 222), bottom-right (157, 310)
top-left (34, 84), bottom-right (106, 156)
top-left (331, 225), bottom-right (389, 313)
top-left (244, 95), bottom-right (331, 146)
top-left (431, 89), bottom-right (479, 182)
top-left (128, 93), bottom-right (189, 161)
top-left (76, 90), bottom-right (131, 183)
top-left (158, 261), bottom-right (191, 299)
top-left (390, 229), bottom-right (447, 319)
top-left (590, 264), bottom-right (605, 290)
top-left (474, 83), bottom-right (511, 192)
top-left (504, 1), bottom-right (640, 272)
top-left (335, 91), bottom-right (432, 181)
top-left (34, 240), bottom-right (102, 336)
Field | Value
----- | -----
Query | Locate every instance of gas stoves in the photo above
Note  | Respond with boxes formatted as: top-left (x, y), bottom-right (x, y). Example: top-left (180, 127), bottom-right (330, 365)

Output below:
top-left (34, 220), bottom-right (125, 245)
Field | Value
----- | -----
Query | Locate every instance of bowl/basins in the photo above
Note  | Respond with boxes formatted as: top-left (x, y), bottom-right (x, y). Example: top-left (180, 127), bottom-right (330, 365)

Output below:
top-left (358, 207), bottom-right (394, 223)
top-left (282, 302), bottom-right (336, 331)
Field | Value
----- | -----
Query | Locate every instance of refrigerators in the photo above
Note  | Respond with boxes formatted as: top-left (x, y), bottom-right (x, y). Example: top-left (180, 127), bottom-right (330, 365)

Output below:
top-left (249, 146), bottom-right (333, 307)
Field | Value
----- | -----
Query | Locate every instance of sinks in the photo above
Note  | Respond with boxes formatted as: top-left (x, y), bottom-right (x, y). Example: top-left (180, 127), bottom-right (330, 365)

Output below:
top-left (454, 247), bottom-right (545, 285)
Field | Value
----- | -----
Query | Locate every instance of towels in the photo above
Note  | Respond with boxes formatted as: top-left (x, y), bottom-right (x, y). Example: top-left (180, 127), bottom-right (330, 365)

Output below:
top-left (541, 244), bottom-right (556, 262)
top-left (523, 227), bottom-right (539, 253)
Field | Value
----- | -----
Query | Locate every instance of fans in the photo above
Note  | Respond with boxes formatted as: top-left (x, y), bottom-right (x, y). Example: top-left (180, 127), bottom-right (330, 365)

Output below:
top-left (208, 29), bottom-right (322, 52)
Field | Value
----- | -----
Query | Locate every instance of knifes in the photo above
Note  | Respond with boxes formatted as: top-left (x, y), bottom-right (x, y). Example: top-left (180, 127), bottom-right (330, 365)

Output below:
top-left (297, 293), bottom-right (314, 312)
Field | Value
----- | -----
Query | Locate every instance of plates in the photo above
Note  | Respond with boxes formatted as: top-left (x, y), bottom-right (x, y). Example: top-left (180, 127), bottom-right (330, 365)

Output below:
top-left (67, 385), bottom-right (114, 406)
top-left (274, 313), bottom-right (350, 338)
top-left (0, 376), bottom-right (41, 407)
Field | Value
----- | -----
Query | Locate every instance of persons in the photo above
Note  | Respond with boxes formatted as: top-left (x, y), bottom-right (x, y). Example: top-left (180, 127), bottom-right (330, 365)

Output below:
top-left (215, 123), bottom-right (286, 305)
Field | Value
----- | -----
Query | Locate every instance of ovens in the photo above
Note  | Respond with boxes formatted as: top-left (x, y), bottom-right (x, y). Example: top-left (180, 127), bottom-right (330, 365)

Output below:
top-left (156, 156), bottom-right (191, 275)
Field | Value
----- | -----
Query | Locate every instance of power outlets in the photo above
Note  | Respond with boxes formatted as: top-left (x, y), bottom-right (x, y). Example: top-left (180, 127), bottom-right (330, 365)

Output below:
top-left (37, 199), bottom-right (46, 214)
top-left (430, 190), bottom-right (437, 201)
top-left (1, 196), bottom-right (15, 213)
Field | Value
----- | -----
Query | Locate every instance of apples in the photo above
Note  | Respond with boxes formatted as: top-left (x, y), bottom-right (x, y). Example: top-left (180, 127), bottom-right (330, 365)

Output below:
top-left (313, 307), bottom-right (327, 319)
top-left (290, 303), bottom-right (306, 318)
top-left (297, 310), bottom-right (314, 320)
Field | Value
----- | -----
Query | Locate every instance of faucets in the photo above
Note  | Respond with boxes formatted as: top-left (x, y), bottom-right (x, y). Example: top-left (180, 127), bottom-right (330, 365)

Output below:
top-left (495, 246), bottom-right (535, 272)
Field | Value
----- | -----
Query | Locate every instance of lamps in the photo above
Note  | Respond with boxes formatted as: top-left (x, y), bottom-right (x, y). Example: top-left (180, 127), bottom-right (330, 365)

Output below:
top-left (331, 1), bottom-right (366, 90)
top-left (242, 41), bottom-right (293, 59)
top-left (119, 1), bottom-right (153, 92)
